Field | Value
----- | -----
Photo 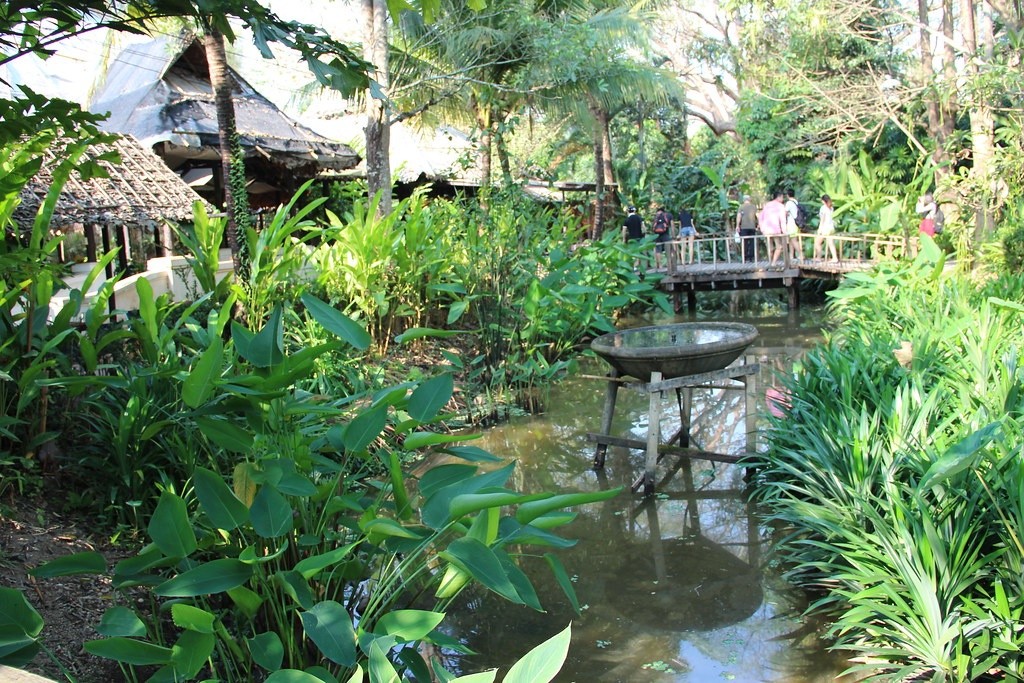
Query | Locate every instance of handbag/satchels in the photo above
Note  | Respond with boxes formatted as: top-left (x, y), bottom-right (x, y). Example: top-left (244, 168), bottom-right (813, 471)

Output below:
top-left (735, 232), bottom-right (740, 243)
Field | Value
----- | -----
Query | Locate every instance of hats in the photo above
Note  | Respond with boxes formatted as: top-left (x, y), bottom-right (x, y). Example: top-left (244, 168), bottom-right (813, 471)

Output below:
top-left (628, 207), bottom-right (636, 211)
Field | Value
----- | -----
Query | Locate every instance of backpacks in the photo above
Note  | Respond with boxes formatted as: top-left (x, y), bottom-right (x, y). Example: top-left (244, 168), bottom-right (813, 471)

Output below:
top-left (928, 202), bottom-right (944, 235)
top-left (653, 212), bottom-right (669, 233)
top-left (789, 200), bottom-right (807, 229)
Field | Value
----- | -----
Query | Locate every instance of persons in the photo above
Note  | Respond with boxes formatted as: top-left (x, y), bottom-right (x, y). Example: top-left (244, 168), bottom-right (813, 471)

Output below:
top-left (811, 194), bottom-right (839, 263)
top-left (653, 205), bottom-right (679, 271)
top-left (735, 194), bottom-right (758, 262)
top-left (784, 188), bottom-right (806, 262)
top-left (758, 192), bottom-right (786, 267)
top-left (622, 206), bottom-right (648, 272)
top-left (676, 209), bottom-right (700, 266)
top-left (916, 191), bottom-right (938, 238)
